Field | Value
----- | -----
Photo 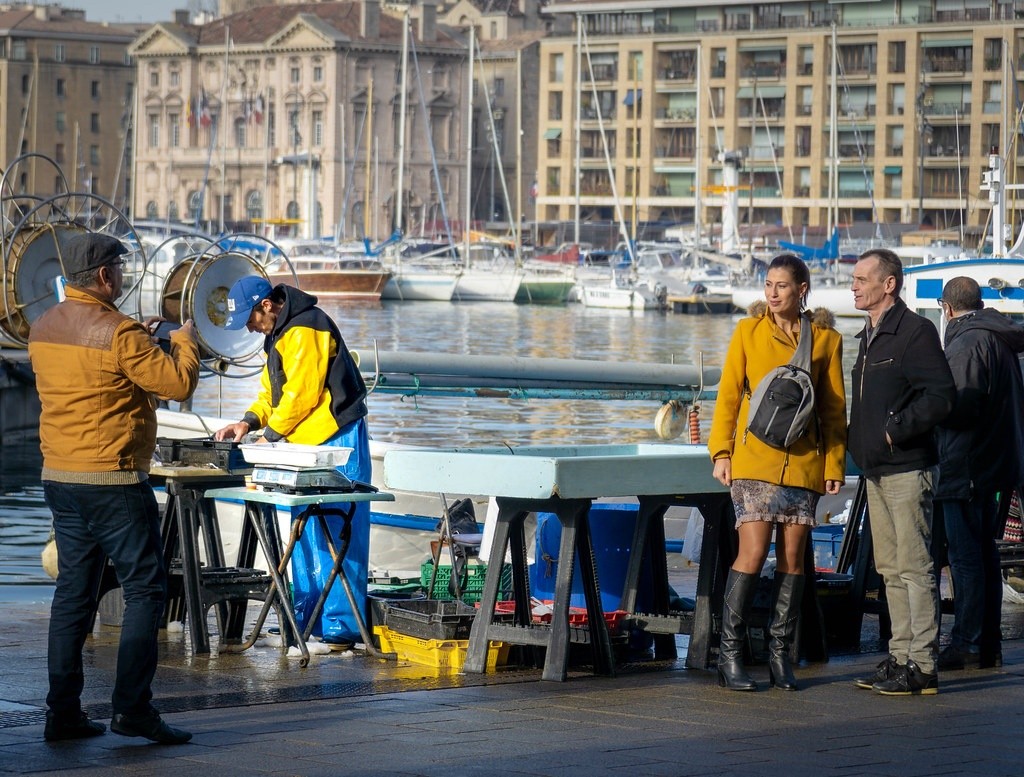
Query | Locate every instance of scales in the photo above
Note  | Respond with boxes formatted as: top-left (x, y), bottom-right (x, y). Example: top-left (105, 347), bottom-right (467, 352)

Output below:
top-left (251, 464), bottom-right (354, 496)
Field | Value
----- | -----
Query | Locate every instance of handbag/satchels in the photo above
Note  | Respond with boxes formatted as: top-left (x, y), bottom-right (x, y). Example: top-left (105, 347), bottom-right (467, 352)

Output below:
top-left (743, 313), bottom-right (819, 451)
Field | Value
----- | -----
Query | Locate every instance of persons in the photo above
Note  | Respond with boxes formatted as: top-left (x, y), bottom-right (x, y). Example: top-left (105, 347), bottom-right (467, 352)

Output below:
top-left (937, 276), bottom-right (1024, 671)
top-left (847, 250), bottom-right (957, 691)
top-left (30, 232), bottom-right (198, 745)
top-left (215, 276), bottom-right (372, 650)
top-left (707, 256), bottom-right (847, 690)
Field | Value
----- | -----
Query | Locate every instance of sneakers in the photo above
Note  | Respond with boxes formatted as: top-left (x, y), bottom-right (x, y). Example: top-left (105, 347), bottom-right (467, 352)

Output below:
top-left (852, 655), bottom-right (908, 689)
top-left (872, 660), bottom-right (939, 695)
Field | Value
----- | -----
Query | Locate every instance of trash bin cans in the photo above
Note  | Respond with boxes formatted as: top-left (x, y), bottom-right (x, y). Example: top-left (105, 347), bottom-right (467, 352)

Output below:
top-left (99, 503), bottom-right (167, 626)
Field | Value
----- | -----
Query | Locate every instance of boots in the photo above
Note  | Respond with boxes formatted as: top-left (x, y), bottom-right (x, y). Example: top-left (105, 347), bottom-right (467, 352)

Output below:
top-left (767, 570), bottom-right (805, 692)
top-left (716, 568), bottom-right (760, 691)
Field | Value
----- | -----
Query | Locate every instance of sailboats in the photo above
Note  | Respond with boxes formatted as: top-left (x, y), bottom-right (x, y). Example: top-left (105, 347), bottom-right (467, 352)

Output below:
top-left (99, 15), bottom-right (908, 317)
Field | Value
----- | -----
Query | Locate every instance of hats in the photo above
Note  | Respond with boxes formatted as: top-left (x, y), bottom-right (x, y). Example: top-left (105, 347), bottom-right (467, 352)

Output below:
top-left (222, 276), bottom-right (274, 331)
top-left (61, 233), bottom-right (129, 275)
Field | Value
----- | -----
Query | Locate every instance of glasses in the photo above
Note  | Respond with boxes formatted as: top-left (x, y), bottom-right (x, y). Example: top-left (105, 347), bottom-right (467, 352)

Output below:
top-left (104, 258), bottom-right (133, 273)
top-left (937, 296), bottom-right (951, 307)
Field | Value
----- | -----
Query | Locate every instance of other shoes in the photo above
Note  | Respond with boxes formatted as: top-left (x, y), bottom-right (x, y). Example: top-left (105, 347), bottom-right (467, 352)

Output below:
top-left (267, 627), bottom-right (281, 637)
top-left (936, 640), bottom-right (1003, 671)
top-left (316, 633), bottom-right (354, 650)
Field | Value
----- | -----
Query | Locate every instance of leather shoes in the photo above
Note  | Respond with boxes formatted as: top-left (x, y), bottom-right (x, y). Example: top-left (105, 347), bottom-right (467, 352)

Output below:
top-left (110, 712), bottom-right (194, 744)
top-left (43, 711), bottom-right (107, 742)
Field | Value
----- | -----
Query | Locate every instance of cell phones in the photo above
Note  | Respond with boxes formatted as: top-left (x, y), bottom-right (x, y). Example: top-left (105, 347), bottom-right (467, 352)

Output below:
top-left (151, 321), bottom-right (184, 342)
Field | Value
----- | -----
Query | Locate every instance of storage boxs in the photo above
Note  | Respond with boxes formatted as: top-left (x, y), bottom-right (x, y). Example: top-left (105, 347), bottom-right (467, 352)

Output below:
top-left (473, 595), bottom-right (629, 628)
top-left (814, 569), bottom-right (857, 651)
top-left (238, 441), bottom-right (356, 467)
top-left (364, 577), bottom-right (425, 652)
top-left (419, 553), bottom-right (514, 605)
top-left (372, 624), bottom-right (504, 670)
top-left (153, 434), bottom-right (249, 470)
top-left (811, 525), bottom-right (880, 590)
top-left (377, 599), bottom-right (516, 640)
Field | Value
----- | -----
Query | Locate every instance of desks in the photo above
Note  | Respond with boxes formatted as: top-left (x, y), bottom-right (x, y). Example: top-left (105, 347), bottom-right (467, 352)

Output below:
top-left (146, 463), bottom-right (296, 656)
top-left (199, 486), bottom-right (399, 667)
